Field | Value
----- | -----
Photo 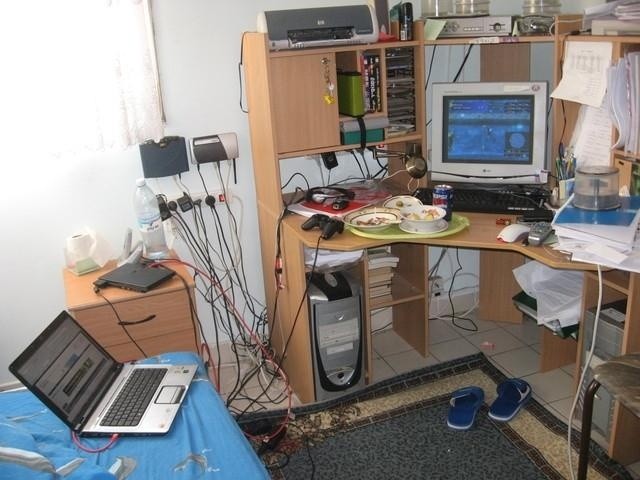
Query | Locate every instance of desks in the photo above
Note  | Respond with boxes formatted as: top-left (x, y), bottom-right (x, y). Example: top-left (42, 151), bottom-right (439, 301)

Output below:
top-left (0, 351), bottom-right (272, 479)
top-left (62, 250), bottom-right (201, 362)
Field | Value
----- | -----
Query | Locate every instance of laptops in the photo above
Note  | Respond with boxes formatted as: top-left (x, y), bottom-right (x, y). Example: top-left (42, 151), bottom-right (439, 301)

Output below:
top-left (9, 311), bottom-right (199, 439)
top-left (96, 263), bottom-right (176, 292)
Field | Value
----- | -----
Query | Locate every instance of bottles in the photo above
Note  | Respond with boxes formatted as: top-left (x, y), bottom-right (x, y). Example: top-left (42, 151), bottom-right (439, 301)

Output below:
top-left (133, 178), bottom-right (170, 262)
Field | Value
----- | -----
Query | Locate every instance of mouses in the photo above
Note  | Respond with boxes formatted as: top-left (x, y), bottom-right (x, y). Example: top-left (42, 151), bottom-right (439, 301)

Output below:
top-left (496, 223), bottom-right (530, 244)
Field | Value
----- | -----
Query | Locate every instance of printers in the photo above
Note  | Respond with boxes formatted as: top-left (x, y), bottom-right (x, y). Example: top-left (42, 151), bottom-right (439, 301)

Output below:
top-left (257, 5), bottom-right (379, 51)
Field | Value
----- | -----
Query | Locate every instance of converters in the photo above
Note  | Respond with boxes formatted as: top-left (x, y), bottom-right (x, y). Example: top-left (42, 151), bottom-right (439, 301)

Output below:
top-left (177, 198), bottom-right (192, 213)
top-left (157, 195), bottom-right (173, 221)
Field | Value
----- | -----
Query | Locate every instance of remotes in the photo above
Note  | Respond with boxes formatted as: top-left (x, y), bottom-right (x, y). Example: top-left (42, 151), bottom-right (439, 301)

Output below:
top-left (528, 221), bottom-right (552, 247)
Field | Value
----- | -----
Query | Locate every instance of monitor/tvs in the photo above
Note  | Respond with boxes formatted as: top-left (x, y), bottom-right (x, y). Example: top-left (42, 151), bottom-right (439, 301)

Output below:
top-left (431, 81), bottom-right (550, 188)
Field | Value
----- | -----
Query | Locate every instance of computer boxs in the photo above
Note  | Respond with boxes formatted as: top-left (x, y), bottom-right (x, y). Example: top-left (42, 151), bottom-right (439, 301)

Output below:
top-left (306, 272), bottom-right (365, 403)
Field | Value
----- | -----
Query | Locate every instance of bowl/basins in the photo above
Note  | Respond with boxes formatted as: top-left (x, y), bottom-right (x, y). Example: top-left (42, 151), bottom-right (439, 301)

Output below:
top-left (398, 204), bottom-right (447, 229)
top-left (342, 207), bottom-right (401, 232)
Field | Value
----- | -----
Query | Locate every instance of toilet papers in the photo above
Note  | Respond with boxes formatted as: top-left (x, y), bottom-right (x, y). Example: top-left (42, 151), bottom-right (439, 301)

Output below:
top-left (67, 225), bottom-right (115, 269)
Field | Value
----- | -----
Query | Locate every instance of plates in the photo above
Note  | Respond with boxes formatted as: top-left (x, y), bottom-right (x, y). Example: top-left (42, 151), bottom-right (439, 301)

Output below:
top-left (383, 195), bottom-right (423, 211)
top-left (398, 219), bottom-right (449, 234)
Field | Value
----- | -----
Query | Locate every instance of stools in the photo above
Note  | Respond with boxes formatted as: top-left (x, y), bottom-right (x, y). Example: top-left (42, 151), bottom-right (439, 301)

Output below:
top-left (578, 352), bottom-right (639, 480)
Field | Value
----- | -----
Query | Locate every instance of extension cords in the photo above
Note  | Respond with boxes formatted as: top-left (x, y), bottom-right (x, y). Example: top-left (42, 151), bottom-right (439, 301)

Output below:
top-left (157, 197), bottom-right (231, 214)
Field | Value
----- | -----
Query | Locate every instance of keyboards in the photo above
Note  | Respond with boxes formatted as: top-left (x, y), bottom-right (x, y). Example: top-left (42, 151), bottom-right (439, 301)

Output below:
top-left (412, 186), bottom-right (550, 216)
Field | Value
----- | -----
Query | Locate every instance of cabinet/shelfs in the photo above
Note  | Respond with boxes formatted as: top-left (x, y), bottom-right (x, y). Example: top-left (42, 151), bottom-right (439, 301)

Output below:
top-left (242, 12), bottom-right (640, 404)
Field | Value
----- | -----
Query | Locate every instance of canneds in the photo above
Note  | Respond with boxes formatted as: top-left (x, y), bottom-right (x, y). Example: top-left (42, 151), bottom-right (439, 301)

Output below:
top-left (432, 184), bottom-right (454, 222)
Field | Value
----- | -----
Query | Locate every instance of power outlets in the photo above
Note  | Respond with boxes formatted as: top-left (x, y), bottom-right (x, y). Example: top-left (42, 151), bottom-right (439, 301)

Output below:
top-left (167, 190), bottom-right (234, 216)
top-left (429, 276), bottom-right (444, 300)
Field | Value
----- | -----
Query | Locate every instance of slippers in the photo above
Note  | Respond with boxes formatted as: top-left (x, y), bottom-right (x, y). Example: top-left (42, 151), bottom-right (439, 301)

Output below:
top-left (447, 386), bottom-right (484, 430)
top-left (488, 378), bottom-right (531, 421)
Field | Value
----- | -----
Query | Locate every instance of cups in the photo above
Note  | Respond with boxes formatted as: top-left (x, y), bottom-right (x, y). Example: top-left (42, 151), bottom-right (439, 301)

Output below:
top-left (556, 177), bottom-right (576, 208)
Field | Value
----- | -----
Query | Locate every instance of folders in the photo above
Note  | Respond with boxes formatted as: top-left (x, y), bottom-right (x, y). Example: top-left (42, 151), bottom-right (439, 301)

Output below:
top-left (512, 291), bottom-right (579, 339)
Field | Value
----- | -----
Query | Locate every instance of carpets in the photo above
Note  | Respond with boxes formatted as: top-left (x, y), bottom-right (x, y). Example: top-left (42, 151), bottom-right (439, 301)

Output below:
top-left (231, 351), bottom-right (634, 480)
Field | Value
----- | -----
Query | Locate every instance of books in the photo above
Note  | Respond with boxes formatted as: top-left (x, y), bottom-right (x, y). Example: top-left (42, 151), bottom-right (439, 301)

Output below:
top-left (582, 0), bottom-right (640, 36)
top-left (365, 247), bottom-right (400, 307)
top-left (358, 55), bottom-right (382, 112)
top-left (513, 270), bottom-right (583, 341)
top-left (550, 189), bottom-right (640, 273)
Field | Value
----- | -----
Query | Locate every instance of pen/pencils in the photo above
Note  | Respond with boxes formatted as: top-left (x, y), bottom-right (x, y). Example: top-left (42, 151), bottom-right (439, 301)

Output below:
top-left (555, 143), bottom-right (576, 180)
